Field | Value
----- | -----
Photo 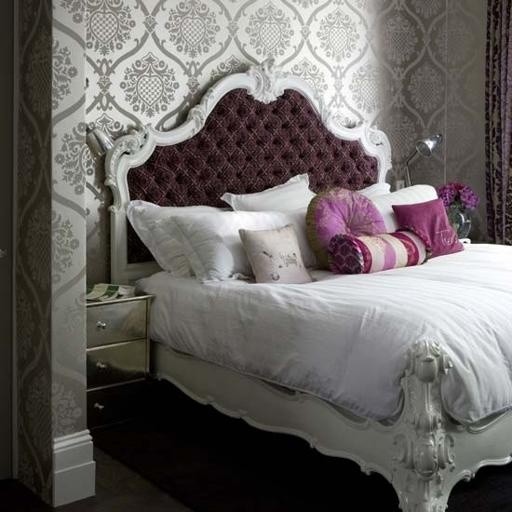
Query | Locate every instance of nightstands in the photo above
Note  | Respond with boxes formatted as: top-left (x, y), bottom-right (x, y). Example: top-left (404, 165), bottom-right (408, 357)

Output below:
top-left (83, 291), bottom-right (155, 429)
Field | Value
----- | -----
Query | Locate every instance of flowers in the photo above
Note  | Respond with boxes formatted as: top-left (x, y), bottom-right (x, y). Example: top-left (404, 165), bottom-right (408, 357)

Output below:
top-left (438, 176), bottom-right (479, 232)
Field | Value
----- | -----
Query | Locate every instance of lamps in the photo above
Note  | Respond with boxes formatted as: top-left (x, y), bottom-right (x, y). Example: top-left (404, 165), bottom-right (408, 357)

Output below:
top-left (400, 132), bottom-right (443, 187)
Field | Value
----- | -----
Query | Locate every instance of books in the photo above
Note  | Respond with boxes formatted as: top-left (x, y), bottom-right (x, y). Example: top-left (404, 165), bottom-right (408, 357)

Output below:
top-left (83, 282), bottom-right (138, 304)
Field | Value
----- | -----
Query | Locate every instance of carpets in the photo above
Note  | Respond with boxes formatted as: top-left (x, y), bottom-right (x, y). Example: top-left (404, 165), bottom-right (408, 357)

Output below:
top-left (86, 425), bottom-right (510, 512)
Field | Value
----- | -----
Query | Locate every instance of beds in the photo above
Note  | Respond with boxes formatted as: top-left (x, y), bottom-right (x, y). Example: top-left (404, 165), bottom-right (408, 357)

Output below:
top-left (86, 59), bottom-right (510, 512)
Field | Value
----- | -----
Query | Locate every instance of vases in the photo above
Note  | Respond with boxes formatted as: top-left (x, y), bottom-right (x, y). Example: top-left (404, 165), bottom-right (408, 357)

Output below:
top-left (448, 208), bottom-right (471, 239)
